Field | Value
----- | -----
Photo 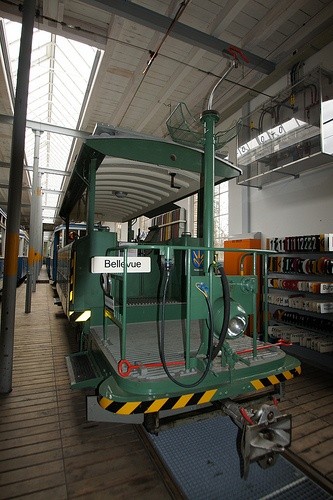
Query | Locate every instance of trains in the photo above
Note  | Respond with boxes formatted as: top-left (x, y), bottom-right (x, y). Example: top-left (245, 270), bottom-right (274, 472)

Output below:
top-left (47, 109), bottom-right (302, 482)
top-left (0, 207), bottom-right (30, 293)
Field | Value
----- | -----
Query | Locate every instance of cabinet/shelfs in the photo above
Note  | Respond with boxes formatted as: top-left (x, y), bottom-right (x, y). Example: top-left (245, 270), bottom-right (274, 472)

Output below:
top-left (265, 252), bottom-right (333, 373)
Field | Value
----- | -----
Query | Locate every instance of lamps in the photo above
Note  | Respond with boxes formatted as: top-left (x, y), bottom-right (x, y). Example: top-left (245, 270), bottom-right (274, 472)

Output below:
top-left (168, 167), bottom-right (181, 191)
